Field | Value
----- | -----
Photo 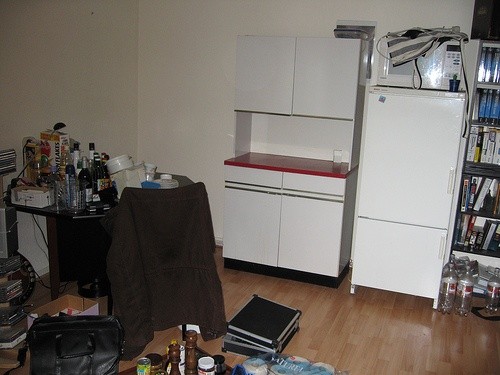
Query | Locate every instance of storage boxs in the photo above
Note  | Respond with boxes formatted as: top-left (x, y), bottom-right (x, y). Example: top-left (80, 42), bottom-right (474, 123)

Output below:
top-left (40, 130), bottom-right (70, 175)
top-left (221, 294), bottom-right (302, 358)
top-left (28, 294), bottom-right (99, 331)
top-left (11, 186), bottom-right (55, 208)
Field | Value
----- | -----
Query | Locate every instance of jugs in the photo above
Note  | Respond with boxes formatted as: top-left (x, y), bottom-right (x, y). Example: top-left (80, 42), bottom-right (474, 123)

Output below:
top-left (104, 154), bottom-right (146, 199)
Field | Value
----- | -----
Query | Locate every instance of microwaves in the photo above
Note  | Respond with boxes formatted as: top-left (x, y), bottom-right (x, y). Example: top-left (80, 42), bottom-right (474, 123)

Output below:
top-left (371, 35), bottom-right (464, 90)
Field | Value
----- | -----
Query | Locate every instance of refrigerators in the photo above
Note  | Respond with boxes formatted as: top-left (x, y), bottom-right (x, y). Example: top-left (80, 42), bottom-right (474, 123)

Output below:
top-left (350, 85), bottom-right (468, 309)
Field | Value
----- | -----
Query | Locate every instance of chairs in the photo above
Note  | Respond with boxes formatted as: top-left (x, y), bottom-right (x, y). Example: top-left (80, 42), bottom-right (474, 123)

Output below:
top-left (5, 315), bottom-right (122, 375)
top-left (108, 182), bottom-right (205, 341)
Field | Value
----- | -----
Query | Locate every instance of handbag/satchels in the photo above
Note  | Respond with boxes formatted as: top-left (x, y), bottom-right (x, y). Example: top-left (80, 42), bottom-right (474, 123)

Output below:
top-left (25, 310), bottom-right (129, 375)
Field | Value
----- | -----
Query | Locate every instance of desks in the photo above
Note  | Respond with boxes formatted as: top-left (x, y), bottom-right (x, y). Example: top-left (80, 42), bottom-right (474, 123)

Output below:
top-left (6, 172), bottom-right (194, 292)
top-left (117, 346), bottom-right (233, 375)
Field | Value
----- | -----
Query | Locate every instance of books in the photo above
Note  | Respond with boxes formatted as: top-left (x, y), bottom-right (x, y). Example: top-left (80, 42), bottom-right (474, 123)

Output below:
top-left (453, 45), bottom-right (500, 251)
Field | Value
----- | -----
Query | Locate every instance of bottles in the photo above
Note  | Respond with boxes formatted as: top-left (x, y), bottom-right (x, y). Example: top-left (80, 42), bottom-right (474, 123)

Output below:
top-left (485, 268), bottom-right (500, 314)
top-left (64, 158), bottom-right (76, 208)
top-left (90, 153), bottom-right (110, 203)
top-left (453, 266), bottom-right (474, 318)
top-left (73, 143), bottom-right (79, 168)
top-left (78, 156), bottom-right (93, 208)
top-left (438, 262), bottom-right (457, 315)
top-left (89, 142), bottom-right (96, 171)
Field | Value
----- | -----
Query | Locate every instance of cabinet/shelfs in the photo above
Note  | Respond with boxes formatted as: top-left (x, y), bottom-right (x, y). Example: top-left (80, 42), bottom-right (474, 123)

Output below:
top-left (440, 41), bottom-right (500, 298)
top-left (222, 35), bottom-right (369, 289)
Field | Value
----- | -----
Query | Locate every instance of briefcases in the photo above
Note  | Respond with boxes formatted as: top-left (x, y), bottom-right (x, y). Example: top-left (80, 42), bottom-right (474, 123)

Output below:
top-left (222, 295), bottom-right (302, 360)
